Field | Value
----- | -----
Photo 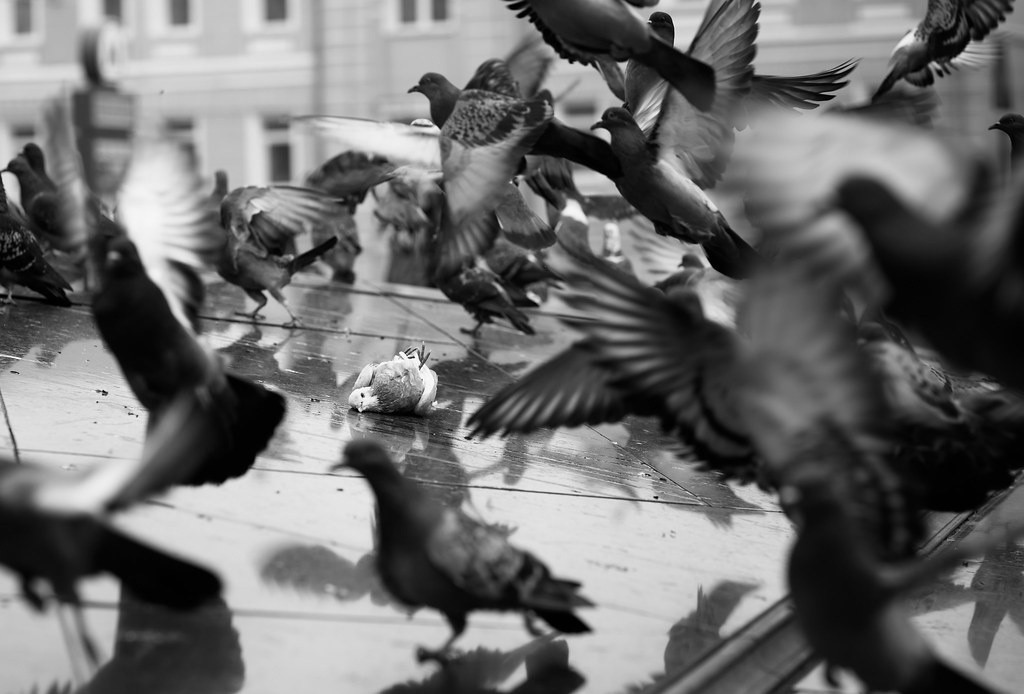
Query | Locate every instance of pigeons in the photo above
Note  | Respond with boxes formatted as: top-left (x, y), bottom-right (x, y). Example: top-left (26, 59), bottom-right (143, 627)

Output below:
top-left (0, 2), bottom-right (1023, 694)
